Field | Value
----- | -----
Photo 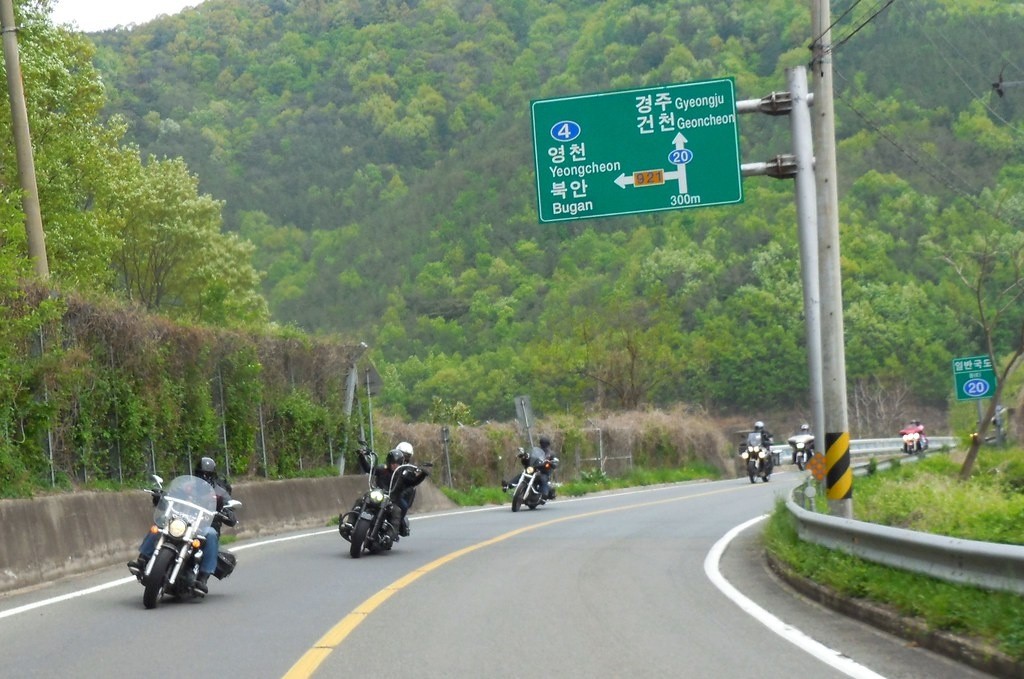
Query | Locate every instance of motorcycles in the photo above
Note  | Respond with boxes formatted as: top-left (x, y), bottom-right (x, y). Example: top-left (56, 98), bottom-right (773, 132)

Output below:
top-left (128, 476), bottom-right (244, 609)
top-left (739, 431), bottom-right (771, 484)
top-left (338, 438), bottom-right (434, 558)
top-left (788, 432), bottom-right (815, 471)
top-left (511, 446), bottom-right (555, 513)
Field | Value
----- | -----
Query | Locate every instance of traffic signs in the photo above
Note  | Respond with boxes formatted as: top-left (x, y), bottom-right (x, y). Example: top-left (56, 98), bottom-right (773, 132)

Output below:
top-left (529, 76), bottom-right (746, 225)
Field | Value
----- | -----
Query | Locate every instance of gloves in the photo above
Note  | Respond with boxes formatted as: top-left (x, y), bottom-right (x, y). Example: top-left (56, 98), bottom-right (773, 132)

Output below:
top-left (225, 509), bottom-right (235, 525)
top-left (151, 488), bottom-right (163, 498)
top-left (421, 468), bottom-right (429, 476)
top-left (356, 449), bottom-right (365, 462)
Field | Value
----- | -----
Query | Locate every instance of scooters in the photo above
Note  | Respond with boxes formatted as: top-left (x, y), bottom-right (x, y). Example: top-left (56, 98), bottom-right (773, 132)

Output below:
top-left (900, 427), bottom-right (928, 456)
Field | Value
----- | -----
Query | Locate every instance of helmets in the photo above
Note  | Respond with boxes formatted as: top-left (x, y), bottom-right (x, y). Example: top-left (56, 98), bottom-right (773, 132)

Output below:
top-left (911, 420), bottom-right (916, 424)
top-left (387, 449), bottom-right (404, 472)
top-left (540, 437), bottom-right (549, 448)
top-left (396, 442), bottom-right (414, 457)
top-left (195, 458), bottom-right (217, 483)
top-left (916, 421), bottom-right (921, 426)
top-left (755, 421), bottom-right (764, 427)
top-left (801, 424), bottom-right (809, 430)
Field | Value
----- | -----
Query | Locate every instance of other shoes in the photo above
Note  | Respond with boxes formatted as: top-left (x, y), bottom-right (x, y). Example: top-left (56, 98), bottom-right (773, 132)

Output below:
top-left (383, 535), bottom-right (391, 542)
top-left (127, 553), bottom-right (149, 571)
top-left (501, 480), bottom-right (508, 492)
top-left (195, 572), bottom-right (209, 593)
top-left (542, 498), bottom-right (546, 505)
top-left (346, 522), bottom-right (353, 528)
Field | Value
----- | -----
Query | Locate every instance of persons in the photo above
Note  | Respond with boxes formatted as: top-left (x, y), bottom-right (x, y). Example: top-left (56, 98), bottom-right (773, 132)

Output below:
top-left (378, 441), bottom-right (414, 518)
top-left (902, 419), bottom-right (921, 453)
top-left (501, 437), bottom-right (558, 506)
top-left (340, 449), bottom-right (421, 542)
top-left (740, 421), bottom-right (773, 477)
top-left (790, 424), bottom-right (815, 465)
top-left (915, 419), bottom-right (920, 426)
top-left (128, 457), bottom-right (236, 597)
